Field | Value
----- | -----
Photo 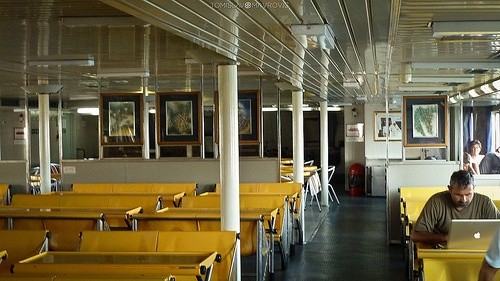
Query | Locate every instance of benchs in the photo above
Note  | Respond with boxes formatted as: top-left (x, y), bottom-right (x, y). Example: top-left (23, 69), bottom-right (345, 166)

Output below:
top-left (0, 183), bottom-right (500, 281)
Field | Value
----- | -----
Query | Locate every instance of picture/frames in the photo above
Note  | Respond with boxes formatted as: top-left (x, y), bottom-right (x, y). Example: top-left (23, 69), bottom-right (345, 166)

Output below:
top-left (214, 89), bottom-right (261, 145)
top-left (403, 95), bottom-right (448, 147)
top-left (156, 90), bottom-right (203, 146)
top-left (99, 91), bottom-right (143, 147)
top-left (373, 110), bottom-right (402, 141)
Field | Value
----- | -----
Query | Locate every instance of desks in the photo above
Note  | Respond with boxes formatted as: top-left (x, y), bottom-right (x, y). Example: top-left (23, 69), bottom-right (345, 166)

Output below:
top-left (281, 166), bottom-right (317, 174)
top-left (415, 243), bottom-right (500, 281)
top-left (10, 251), bottom-right (222, 281)
top-left (0, 205), bottom-right (144, 231)
top-left (195, 192), bottom-right (300, 256)
top-left (281, 158), bottom-right (294, 164)
top-left (40, 192), bottom-right (186, 208)
top-left (151, 207), bottom-right (280, 281)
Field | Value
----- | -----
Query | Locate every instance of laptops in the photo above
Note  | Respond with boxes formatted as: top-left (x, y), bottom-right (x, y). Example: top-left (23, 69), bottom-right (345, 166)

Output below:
top-left (438, 219), bottom-right (500, 251)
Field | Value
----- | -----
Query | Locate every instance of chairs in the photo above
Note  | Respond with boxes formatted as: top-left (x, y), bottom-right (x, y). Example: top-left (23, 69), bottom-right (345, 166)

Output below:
top-left (304, 160), bottom-right (340, 212)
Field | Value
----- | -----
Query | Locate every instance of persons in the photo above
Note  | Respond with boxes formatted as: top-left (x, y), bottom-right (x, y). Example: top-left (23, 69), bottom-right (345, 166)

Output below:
top-left (478, 228), bottom-right (500, 281)
top-left (463, 140), bottom-right (500, 174)
top-left (382, 121), bottom-right (399, 136)
top-left (410, 170), bottom-right (500, 249)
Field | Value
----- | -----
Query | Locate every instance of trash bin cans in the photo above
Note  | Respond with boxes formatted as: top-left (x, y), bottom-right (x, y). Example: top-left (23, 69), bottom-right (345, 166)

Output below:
top-left (348, 164), bottom-right (364, 196)
